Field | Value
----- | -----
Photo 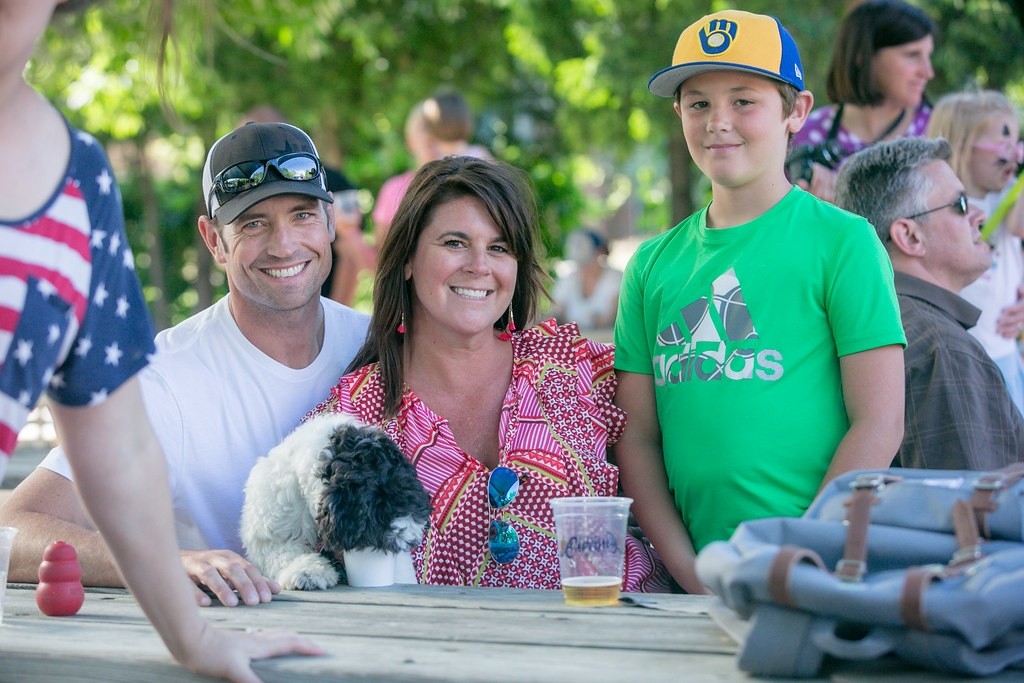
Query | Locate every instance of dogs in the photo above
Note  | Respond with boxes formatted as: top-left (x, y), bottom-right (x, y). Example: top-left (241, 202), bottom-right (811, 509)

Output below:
top-left (238, 410), bottom-right (432, 591)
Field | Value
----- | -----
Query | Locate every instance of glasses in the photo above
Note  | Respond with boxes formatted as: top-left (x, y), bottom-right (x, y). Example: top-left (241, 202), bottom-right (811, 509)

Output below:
top-left (970, 138), bottom-right (1023, 162)
top-left (884, 191), bottom-right (971, 241)
top-left (208, 150), bottom-right (328, 225)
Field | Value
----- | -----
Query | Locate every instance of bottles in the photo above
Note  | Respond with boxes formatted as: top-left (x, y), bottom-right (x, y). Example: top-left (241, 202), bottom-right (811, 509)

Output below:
top-left (36, 540), bottom-right (84, 616)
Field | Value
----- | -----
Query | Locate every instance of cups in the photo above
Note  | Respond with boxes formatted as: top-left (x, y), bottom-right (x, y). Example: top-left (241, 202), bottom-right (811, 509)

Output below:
top-left (0, 526), bottom-right (18, 625)
top-left (549, 498), bottom-right (634, 608)
top-left (332, 190), bottom-right (360, 225)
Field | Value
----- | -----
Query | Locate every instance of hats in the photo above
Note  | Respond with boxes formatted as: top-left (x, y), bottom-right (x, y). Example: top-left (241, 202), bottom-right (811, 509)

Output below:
top-left (647, 7), bottom-right (803, 95)
top-left (202, 120), bottom-right (335, 225)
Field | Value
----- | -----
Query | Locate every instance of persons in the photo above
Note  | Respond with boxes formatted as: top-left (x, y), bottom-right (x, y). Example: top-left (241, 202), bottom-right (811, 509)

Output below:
top-left (610, 10), bottom-right (907, 597)
top-left (244, 0), bottom-right (1024, 475)
top-left (0, 120), bottom-right (373, 607)
top-left (0, 0), bottom-right (327, 683)
top-left (295, 152), bottom-right (688, 594)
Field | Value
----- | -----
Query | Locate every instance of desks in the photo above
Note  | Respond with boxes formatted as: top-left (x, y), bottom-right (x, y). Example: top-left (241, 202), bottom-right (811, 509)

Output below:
top-left (0, 574), bottom-right (754, 683)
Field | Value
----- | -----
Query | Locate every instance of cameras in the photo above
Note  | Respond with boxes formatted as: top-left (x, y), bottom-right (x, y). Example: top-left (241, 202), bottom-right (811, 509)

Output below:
top-left (785, 139), bottom-right (845, 184)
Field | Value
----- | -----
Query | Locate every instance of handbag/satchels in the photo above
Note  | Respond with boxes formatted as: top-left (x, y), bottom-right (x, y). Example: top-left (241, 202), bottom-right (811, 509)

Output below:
top-left (723, 458), bottom-right (1024, 683)
